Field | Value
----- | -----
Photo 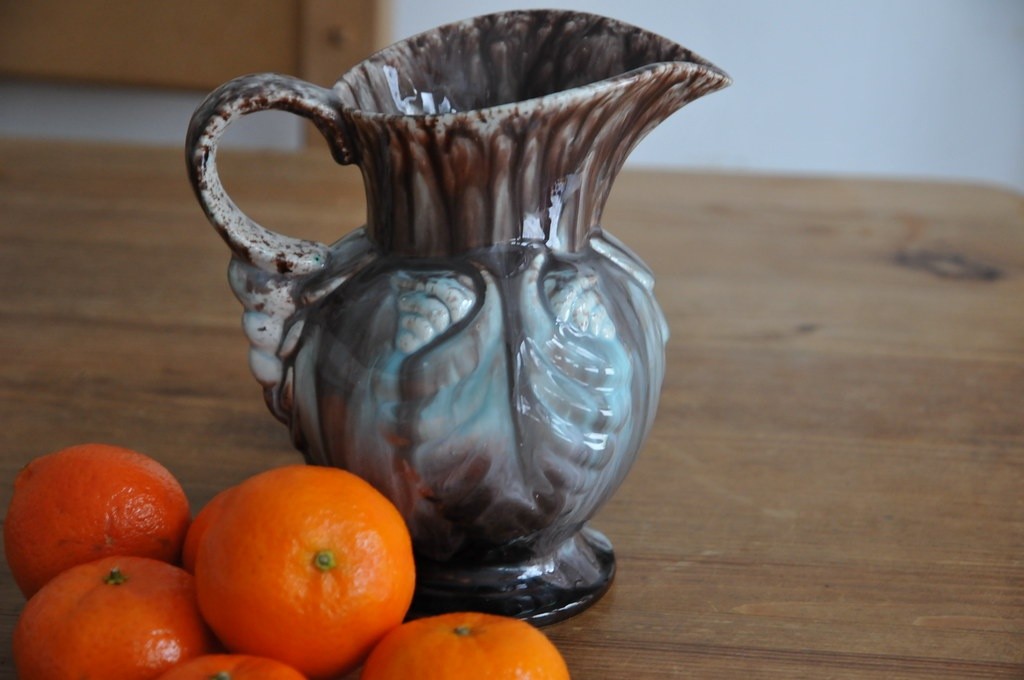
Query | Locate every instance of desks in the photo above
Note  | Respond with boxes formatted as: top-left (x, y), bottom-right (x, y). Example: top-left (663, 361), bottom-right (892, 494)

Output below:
top-left (0, 129), bottom-right (1023, 680)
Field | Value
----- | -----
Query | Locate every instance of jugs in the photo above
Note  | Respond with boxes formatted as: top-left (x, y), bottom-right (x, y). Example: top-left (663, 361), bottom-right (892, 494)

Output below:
top-left (185, 7), bottom-right (737, 626)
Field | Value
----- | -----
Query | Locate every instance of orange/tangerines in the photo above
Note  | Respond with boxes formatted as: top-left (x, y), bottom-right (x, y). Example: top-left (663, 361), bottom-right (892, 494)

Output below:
top-left (6, 446), bottom-right (578, 679)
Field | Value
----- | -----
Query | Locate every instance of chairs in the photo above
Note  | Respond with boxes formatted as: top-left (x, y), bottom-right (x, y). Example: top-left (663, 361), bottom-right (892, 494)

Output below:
top-left (0, 0), bottom-right (393, 147)
